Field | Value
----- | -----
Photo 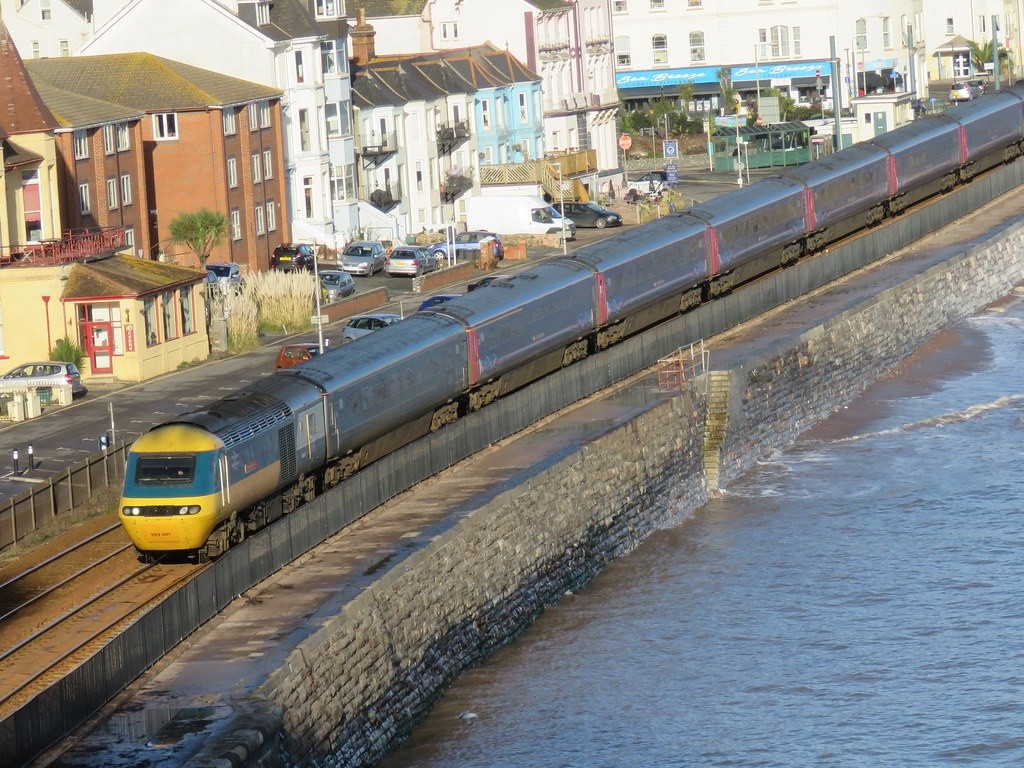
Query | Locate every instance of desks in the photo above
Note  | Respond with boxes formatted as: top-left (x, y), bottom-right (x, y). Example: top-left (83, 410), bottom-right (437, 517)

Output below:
top-left (38, 238), bottom-right (66, 257)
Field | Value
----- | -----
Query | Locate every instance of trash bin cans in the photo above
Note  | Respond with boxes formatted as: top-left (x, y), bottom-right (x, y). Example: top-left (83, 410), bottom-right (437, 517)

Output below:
top-left (479, 242), bottom-right (494, 269)
top-left (35, 385), bottom-right (52, 404)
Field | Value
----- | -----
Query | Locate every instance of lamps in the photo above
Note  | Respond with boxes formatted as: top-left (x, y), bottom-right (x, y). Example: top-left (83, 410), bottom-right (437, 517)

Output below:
top-left (199, 290), bottom-right (206, 300)
top-left (140, 308), bottom-right (147, 317)
top-left (160, 302), bottom-right (165, 309)
top-left (68, 317), bottom-right (73, 326)
top-left (179, 297), bottom-right (184, 304)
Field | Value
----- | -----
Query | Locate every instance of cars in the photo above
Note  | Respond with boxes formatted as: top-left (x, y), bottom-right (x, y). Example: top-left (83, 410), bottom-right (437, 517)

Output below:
top-left (426, 230), bottom-right (504, 261)
top-left (551, 200), bottom-right (624, 228)
top-left (465, 274), bottom-right (507, 292)
top-left (274, 343), bottom-right (334, 371)
top-left (627, 169), bottom-right (677, 203)
top-left (383, 246), bottom-right (439, 278)
top-left (338, 241), bottom-right (386, 278)
top-left (415, 293), bottom-right (462, 312)
top-left (340, 314), bottom-right (403, 347)
top-left (949, 82), bottom-right (980, 102)
top-left (317, 269), bottom-right (355, 302)
top-left (967, 79), bottom-right (988, 94)
top-left (0, 361), bottom-right (83, 395)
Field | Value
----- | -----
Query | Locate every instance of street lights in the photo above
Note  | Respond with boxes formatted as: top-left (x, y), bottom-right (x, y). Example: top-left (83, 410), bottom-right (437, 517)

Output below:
top-left (851, 37), bottom-right (860, 97)
top-left (740, 141), bottom-right (751, 183)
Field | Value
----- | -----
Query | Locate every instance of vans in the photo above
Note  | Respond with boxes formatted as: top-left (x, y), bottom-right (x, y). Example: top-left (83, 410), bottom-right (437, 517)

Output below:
top-left (465, 195), bottom-right (577, 242)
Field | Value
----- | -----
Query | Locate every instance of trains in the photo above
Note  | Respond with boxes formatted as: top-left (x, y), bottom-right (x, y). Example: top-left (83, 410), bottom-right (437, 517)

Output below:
top-left (117, 81), bottom-right (1024, 564)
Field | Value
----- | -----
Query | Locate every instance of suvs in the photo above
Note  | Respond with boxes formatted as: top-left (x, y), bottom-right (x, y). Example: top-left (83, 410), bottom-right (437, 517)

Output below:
top-left (204, 263), bottom-right (244, 297)
top-left (268, 244), bottom-right (319, 275)
